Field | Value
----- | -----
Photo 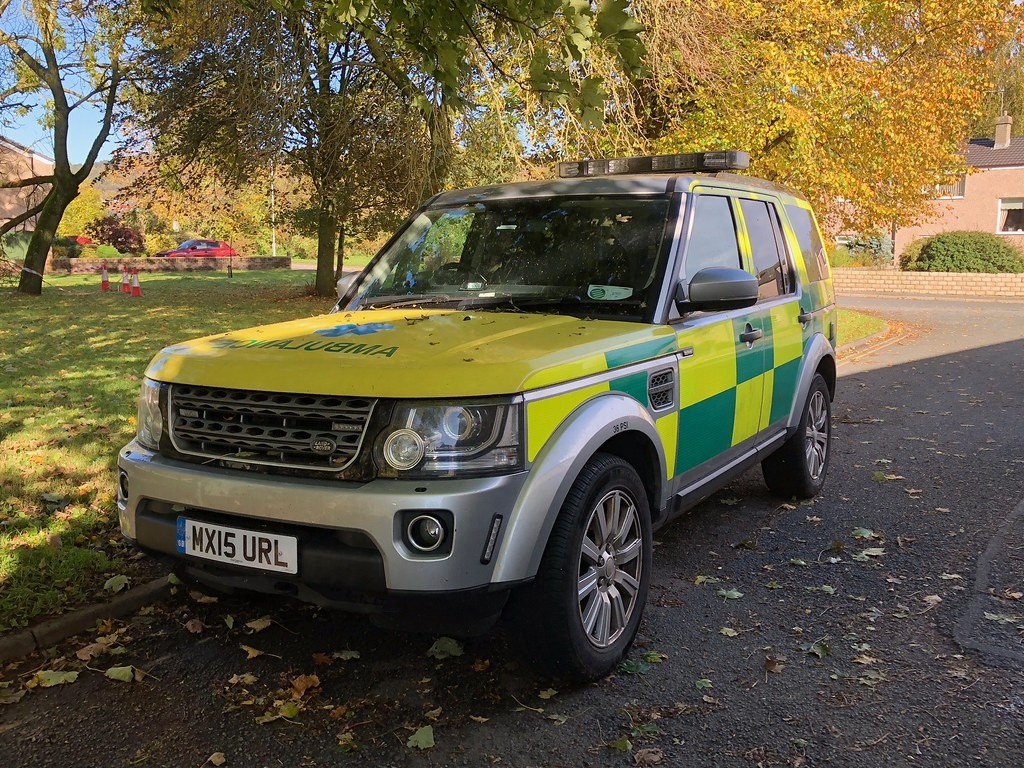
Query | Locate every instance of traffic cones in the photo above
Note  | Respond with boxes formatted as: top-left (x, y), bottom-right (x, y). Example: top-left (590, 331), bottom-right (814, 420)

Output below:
top-left (120, 265), bottom-right (131, 293)
top-left (130, 267), bottom-right (143, 296)
top-left (101, 265), bottom-right (112, 292)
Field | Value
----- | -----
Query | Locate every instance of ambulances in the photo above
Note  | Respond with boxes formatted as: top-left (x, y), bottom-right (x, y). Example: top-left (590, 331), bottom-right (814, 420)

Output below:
top-left (119, 151), bottom-right (839, 688)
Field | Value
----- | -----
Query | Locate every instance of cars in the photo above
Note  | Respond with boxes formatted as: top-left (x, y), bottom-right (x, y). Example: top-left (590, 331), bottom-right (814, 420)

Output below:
top-left (154, 240), bottom-right (237, 257)
